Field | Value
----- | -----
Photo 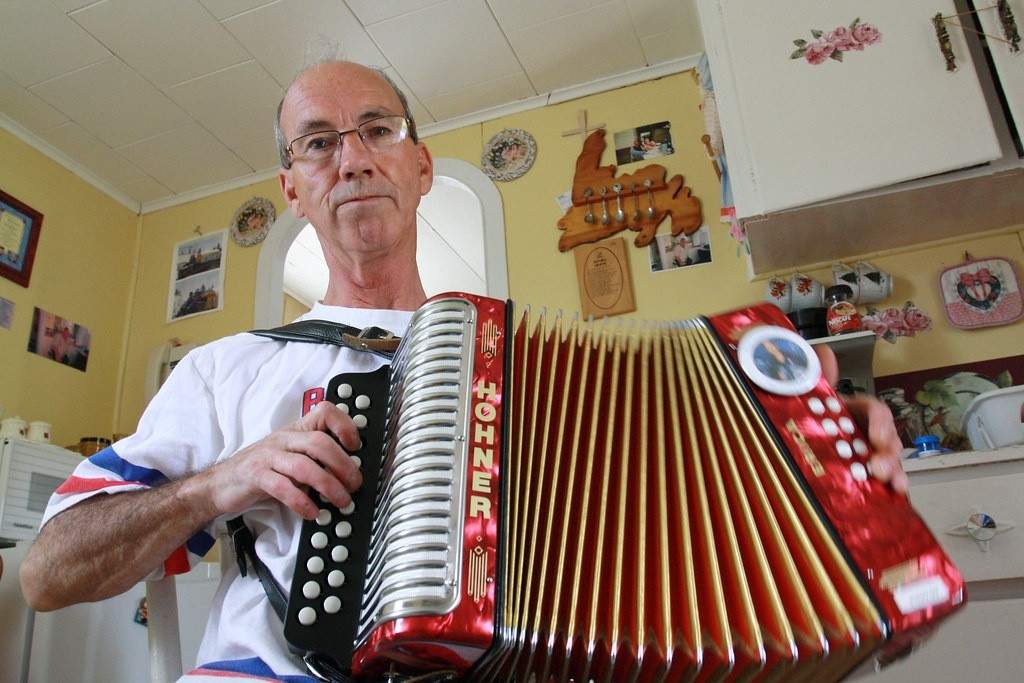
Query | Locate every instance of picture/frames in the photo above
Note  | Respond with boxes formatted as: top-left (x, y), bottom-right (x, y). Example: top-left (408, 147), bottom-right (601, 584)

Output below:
top-left (0, 189), bottom-right (44, 288)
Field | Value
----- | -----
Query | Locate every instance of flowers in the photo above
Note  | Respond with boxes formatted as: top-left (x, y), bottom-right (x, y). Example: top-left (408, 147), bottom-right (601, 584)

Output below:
top-left (860, 301), bottom-right (932, 345)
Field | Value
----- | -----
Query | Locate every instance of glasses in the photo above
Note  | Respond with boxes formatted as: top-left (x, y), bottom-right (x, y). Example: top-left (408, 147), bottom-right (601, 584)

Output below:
top-left (285, 115), bottom-right (410, 165)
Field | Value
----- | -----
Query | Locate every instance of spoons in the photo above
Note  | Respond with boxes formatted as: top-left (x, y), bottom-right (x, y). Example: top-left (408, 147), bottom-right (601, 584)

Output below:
top-left (600, 185), bottom-right (609, 226)
top-left (644, 178), bottom-right (656, 218)
top-left (584, 187), bottom-right (594, 223)
top-left (629, 182), bottom-right (640, 220)
top-left (613, 182), bottom-right (625, 222)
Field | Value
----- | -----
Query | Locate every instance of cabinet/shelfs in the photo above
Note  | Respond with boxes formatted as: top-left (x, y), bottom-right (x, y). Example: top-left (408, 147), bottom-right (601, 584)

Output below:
top-left (696, 0), bottom-right (1024, 282)
top-left (850, 440), bottom-right (1024, 683)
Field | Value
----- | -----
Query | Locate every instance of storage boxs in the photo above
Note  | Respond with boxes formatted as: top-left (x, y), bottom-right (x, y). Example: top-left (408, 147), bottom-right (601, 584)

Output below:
top-left (961, 385), bottom-right (1024, 449)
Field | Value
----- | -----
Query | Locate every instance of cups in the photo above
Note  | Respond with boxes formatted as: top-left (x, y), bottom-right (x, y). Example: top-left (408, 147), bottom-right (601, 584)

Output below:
top-left (30, 421), bottom-right (52, 445)
top-left (765, 260), bottom-right (894, 314)
top-left (1, 415), bottom-right (26, 438)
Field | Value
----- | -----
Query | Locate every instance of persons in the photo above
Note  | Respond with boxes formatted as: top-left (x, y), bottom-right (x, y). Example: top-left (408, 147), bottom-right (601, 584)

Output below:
top-left (53, 327), bottom-right (79, 365)
top-left (20, 62), bottom-right (906, 683)
top-left (632, 137), bottom-right (662, 161)
top-left (672, 238), bottom-right (700, 267)
top-left (182, 288), bottom-right (197, 313)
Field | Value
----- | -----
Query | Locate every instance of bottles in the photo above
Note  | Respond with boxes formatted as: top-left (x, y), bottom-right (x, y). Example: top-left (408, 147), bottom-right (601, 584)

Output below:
top-left (825, 285), bottom-right (867, 336)
top-left (914, 435), bottom-right (941, 458)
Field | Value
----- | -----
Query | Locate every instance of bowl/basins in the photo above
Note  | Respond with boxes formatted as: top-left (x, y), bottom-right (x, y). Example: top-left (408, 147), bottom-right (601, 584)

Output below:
top-left (787, 307), bottom-right (828, 340)
